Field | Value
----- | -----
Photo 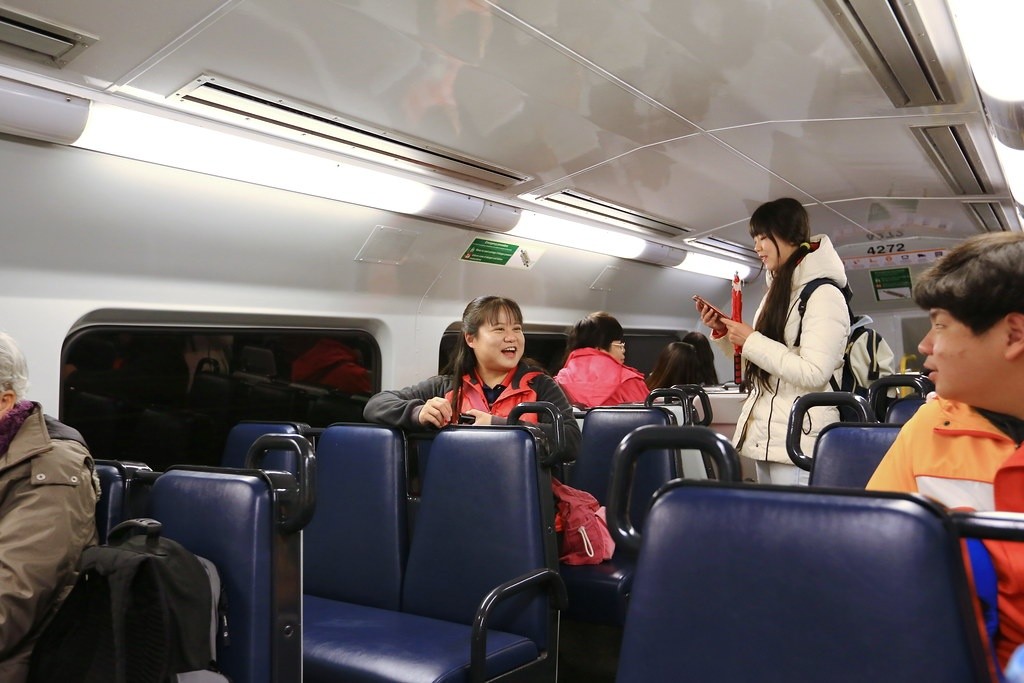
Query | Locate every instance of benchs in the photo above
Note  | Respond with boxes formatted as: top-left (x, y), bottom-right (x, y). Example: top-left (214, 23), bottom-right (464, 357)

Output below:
top-left (27, 370), bottom-right (1023, 683)
top-left (1, 0), bottom-right (1024, 283)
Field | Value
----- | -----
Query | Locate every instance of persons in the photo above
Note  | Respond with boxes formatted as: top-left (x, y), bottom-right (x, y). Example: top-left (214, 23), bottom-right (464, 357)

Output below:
top-left (694, 197), bottom-right (850, 489)
top-left (0, 351), bottom-right (102, 683)
top-left (363, 295), bottom-right (583, 464)
top-left (645, 330), bottom-right (718, 394)
top-left (861, 229), bottom-right (1024, 683)
top-left (290, 333), bottom-right (372, 395)
top-left (552, 310), bottom-right (660, 410)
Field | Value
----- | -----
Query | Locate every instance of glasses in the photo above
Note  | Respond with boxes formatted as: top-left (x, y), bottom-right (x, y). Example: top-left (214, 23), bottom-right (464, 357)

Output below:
top-left (612, 343), bottom-right (625, 351)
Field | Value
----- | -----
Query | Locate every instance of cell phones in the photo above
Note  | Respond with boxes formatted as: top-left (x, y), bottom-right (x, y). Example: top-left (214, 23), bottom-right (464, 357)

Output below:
top-left (692, 294), bottom-right (730, 321)
top-left (457, 413), bottom-right (476, 425)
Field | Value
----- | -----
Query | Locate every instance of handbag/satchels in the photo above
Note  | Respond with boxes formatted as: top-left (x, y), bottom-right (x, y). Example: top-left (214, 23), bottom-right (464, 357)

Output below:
top-left (552, 485), bottom-right (617, 567)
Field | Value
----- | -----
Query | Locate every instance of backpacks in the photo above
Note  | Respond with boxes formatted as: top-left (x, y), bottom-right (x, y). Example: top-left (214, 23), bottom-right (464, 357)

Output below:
top-left (793, 276), bottom-right (896, 425)
top-left (26, 518), bottom-right (231, 683)
